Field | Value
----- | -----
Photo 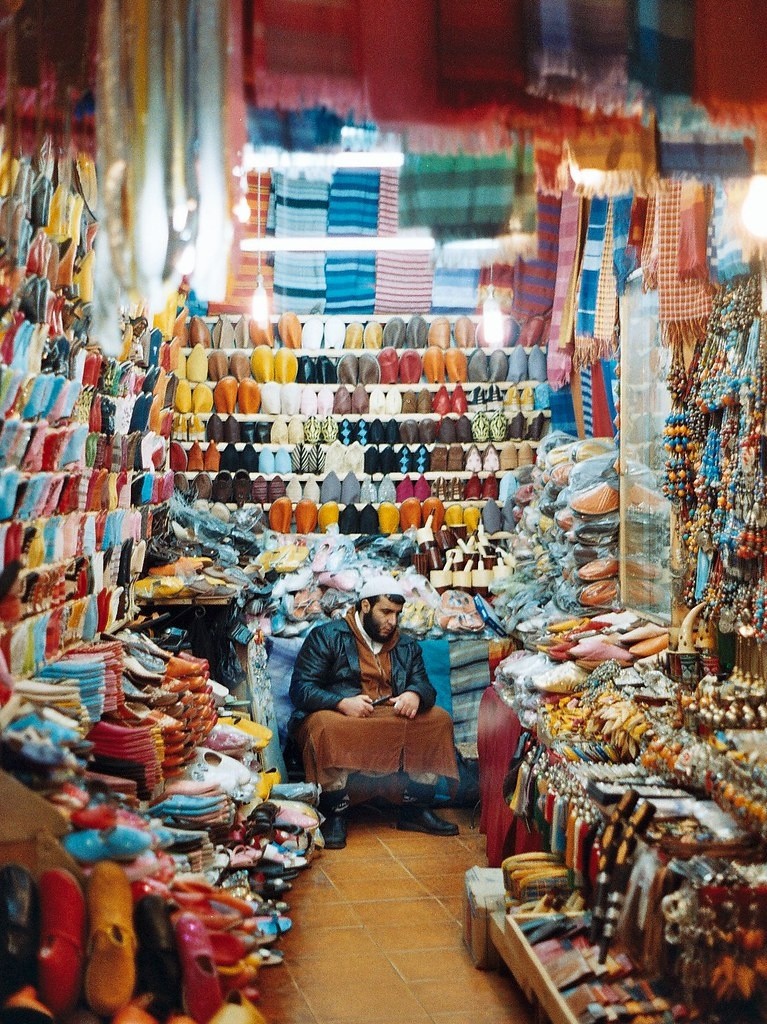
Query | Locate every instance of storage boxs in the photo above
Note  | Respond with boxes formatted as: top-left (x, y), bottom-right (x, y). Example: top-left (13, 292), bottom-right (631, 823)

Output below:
top-left (463, 865), bottom-right (507, 970)
top-left (489, 911), bottom-right (586, 1024)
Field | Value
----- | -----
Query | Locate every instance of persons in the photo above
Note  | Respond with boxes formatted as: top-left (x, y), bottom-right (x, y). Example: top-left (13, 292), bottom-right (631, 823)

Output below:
top-left (285, 575), bottom-right (461, 849)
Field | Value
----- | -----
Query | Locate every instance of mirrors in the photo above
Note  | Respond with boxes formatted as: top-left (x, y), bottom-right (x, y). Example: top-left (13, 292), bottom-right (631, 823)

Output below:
top-left (618, 293), bottom-right (674, 626)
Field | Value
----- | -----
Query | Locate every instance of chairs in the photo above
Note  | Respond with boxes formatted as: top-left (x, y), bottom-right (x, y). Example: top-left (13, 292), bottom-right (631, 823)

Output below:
top-left (455, 730), bottom-right (533, 829)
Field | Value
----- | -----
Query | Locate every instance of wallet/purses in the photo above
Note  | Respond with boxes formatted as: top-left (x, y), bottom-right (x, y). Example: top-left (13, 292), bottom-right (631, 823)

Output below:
top-left (517, 909), bottom-right (722, 1024)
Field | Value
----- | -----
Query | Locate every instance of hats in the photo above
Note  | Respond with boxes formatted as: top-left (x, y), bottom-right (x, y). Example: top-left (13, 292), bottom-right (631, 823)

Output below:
top-left (359, 575), bottom-right (403, 602)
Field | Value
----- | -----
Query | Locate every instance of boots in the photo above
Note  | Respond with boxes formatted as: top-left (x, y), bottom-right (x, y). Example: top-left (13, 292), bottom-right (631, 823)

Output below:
top-left (319, 788), bottom-right (350, 849)
top-left (396, 779), bottom-right (459, 836)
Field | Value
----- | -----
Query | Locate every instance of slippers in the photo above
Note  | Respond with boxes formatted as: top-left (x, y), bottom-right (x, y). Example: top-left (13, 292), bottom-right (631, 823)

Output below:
top-left (311, 317), bottom-right (684, 734)
top-left (0, 125), bottom-right (324, 1024)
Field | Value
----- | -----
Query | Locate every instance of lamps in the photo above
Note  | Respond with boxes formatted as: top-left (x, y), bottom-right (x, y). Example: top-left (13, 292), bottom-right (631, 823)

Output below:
top-left (483, 264), bottom-right (504, 345)
top-left (250, 173), bottom-right (270, 330)
